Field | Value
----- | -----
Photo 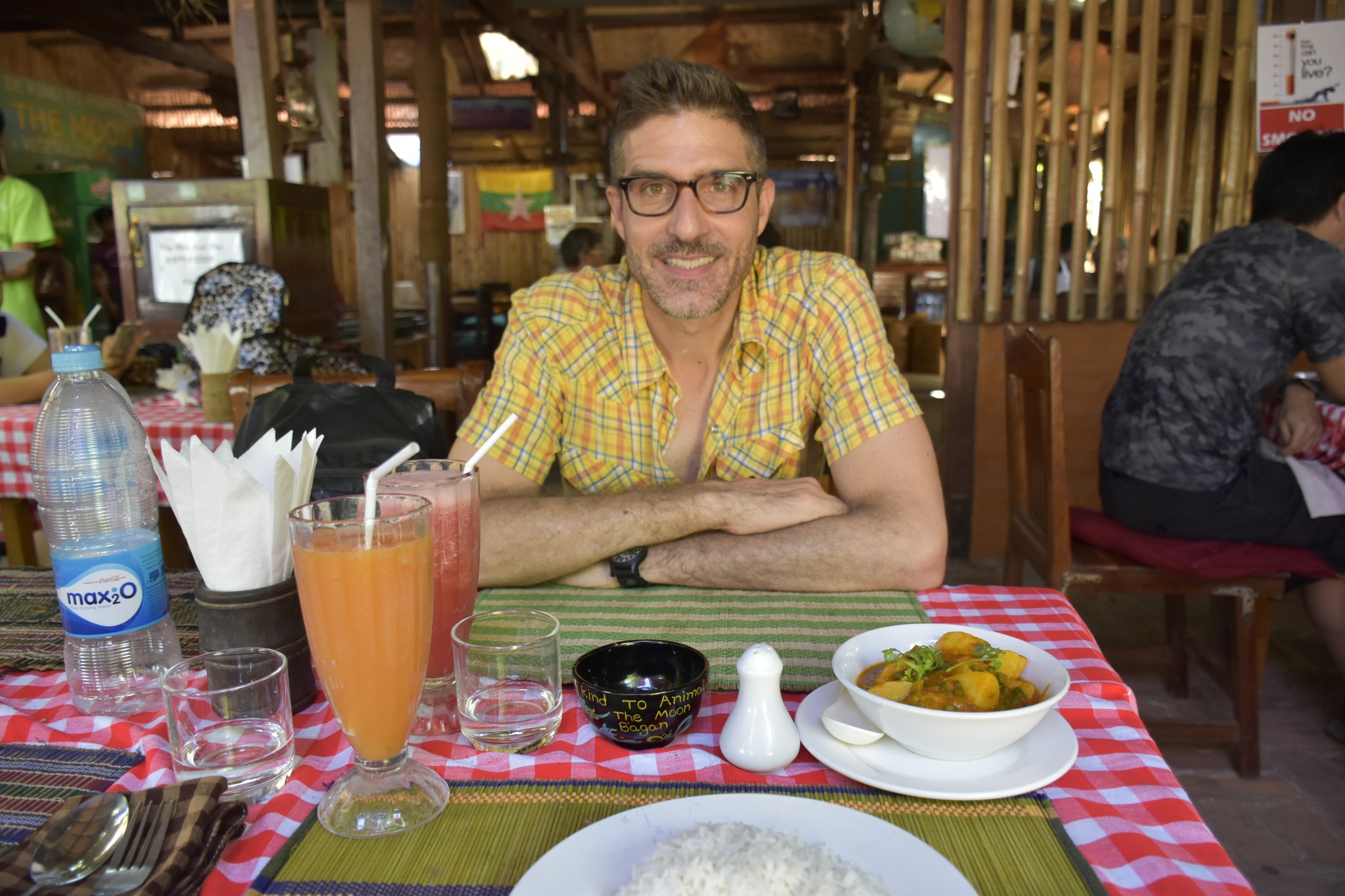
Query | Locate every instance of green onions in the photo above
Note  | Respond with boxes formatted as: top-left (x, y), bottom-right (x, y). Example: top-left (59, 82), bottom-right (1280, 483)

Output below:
top-left (938, 665), bottom-right (1028, 712)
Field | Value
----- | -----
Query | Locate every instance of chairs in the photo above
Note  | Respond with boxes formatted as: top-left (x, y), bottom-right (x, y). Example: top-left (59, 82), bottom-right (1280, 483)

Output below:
top-left (1002, 320), bottom-right (1290, 778)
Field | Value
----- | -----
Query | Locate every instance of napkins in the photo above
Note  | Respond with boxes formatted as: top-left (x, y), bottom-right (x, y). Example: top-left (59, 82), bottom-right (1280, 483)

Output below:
top-left (176, 319), bottom-right (243, 375)
top-left (142, 426), bottom-right (328, 589)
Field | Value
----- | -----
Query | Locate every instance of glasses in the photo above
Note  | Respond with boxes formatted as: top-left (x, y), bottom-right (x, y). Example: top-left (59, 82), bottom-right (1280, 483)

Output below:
top-left (617, 171), bottom-right (766, 216)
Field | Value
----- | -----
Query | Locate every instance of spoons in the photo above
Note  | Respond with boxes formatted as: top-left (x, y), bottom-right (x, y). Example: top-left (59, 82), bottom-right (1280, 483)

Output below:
top-left (22, 790), bottom-right (129, 896)
top-left (820, 686), bottom-right (884, 746)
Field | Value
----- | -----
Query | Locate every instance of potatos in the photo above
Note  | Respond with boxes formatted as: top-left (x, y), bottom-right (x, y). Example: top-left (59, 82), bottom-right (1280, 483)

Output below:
top-left (932, 631), bottom-right (990, 654)
top-left (866, 681), bottom-right (913, 701)
top-left (876, 644), bottom-right (924, 683)
top-left (983, 650), bottom-right (1028, 678)
top-left (945, 671), bottom-right (1000, 708)
top-left (949, 655), bottom-right (988, 674)
top-left (1002, 679), bottom-right (1036, 700)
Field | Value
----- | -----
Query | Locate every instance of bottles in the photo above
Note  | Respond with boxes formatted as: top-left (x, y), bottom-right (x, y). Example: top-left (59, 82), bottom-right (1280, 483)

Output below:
top-left (30, 347), bottom-right (188, 717)
top-left (719, 643), bottom-right (802, 774)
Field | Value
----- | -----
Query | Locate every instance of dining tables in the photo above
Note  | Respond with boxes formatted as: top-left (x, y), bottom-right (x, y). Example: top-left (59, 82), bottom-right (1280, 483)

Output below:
top-left (0, 571), bottom-right (1257, 896)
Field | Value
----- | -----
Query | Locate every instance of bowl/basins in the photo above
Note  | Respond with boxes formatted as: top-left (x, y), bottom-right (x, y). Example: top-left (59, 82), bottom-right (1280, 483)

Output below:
top-left (832, 623), bottom-right (1070, 761)
top-left (571, 640), bottom-right (710, 752)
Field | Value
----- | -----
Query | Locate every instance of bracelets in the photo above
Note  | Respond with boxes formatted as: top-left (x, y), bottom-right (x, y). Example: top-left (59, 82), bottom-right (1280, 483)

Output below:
top-left (1278, 368), bottom-right (1325, 398)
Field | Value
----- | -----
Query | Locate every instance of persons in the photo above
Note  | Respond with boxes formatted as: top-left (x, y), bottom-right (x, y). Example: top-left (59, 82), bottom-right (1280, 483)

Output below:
top-left (0, 110), bottom-right (129, 405)
top-left (1002, 220), bottom-right (1093, 296)
top-left (1098, 127), bottom-right (1345, 678)
top-left (446, 54), bottom-right (949, 591)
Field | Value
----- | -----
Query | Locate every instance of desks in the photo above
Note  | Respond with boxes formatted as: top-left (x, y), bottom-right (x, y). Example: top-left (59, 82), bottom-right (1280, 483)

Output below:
top-left (0, 388), bottom-right (235, 572)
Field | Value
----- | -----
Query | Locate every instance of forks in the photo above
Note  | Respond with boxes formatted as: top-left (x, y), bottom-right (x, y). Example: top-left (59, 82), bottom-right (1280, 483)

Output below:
top-left (93, 799), bottom-right (175, 896)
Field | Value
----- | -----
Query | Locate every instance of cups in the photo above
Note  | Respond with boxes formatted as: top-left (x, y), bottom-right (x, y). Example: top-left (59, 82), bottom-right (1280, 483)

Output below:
top-left (193, 571), bottom-right (318, 722)
top-left (159, 647), bottom-right (296, 807)
top-left (287, 494), bottom-right (450, 839)
top-left (449, 608), bottom-right (563, 755)
top-left (199, 373), bottom-right (232, 422)
top-left (362, 458), bottom-right (480, 734)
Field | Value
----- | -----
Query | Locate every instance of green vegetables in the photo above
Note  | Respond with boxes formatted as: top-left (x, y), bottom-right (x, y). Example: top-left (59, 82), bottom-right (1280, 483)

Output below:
top-left (882, 642), bottom-right (1008, 682)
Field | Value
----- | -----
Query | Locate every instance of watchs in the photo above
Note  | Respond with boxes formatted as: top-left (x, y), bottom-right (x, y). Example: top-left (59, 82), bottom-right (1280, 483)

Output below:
top-left (607, 548), bottom-right (649, 591)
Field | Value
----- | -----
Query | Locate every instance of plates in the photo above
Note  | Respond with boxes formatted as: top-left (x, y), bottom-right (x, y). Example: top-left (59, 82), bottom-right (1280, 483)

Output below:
top-left (508, 793), bottom-right (980, 896)
top-left (794, 680), bottom-right (1079, 801)
